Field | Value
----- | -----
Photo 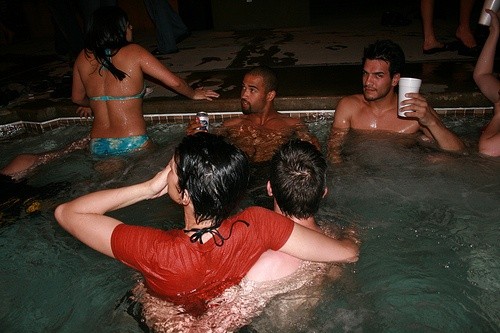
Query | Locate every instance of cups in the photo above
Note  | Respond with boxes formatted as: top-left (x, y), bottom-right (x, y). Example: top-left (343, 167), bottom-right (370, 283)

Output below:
top-left (478, 0), bottom-right (500, 27)
top-left (398, 77), bottom-right (422, 117)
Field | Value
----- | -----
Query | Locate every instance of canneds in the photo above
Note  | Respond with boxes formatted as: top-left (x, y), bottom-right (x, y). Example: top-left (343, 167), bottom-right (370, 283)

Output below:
top-left (195, 112), bottom-right (209, 132)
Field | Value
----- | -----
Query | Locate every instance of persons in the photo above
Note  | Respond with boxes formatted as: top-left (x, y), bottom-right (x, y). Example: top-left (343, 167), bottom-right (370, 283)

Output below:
top-left (332, 39), bottom-right (466, 153)
top-left (143, 0), bottom-right (192, 56)
top-left (185, 65), bottom-right (323, 163)
top-left (51, 132), bottom-right (361, 333)
top-left (71, 2), bottom-right (220, 157)
top-left (420, 0), bottom-right (483, 54)
top-left (0, 133), bottom-right (91, 175)
top-left (472, 9), bottom-right (500, 157)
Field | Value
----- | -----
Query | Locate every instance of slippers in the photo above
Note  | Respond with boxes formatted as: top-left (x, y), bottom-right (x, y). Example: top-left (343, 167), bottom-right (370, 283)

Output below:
top-left (423, 40), bottom-right (454, 55)
